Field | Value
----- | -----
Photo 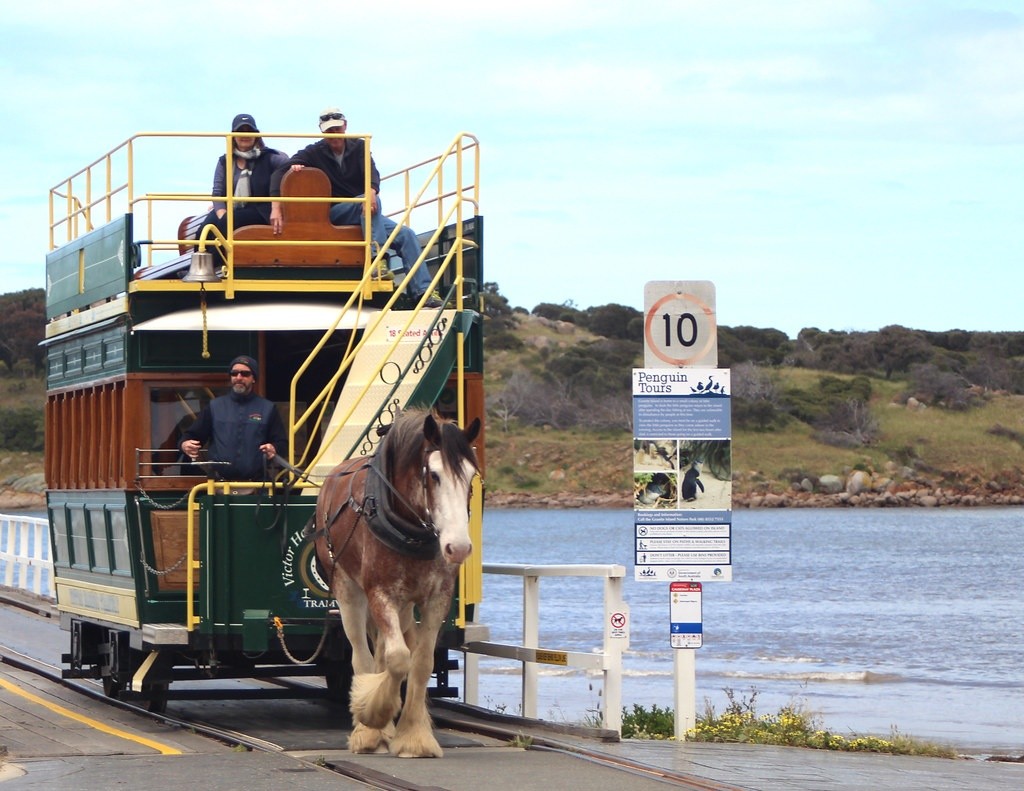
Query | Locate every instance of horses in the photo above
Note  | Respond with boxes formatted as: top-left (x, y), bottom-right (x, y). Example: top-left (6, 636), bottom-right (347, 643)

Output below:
top-left (316, 406), bottom-right (484, 759)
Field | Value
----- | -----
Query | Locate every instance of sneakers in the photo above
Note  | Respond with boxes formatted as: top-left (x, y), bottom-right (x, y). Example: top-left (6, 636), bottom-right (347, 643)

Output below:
top-left (418, 288), bottom-right (453, 310)
top-left (370, 258), bottom-right (395, 280)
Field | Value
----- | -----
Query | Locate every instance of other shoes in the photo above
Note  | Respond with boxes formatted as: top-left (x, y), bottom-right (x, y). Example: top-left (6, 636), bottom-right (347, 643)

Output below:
top-left (177, 270), bottom-right (188, 279)
top-left (214, 267), bottom-right (225, 278)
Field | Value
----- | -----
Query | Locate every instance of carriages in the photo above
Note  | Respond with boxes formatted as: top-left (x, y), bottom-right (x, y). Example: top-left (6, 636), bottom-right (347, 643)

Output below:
top-left (39, 133), bottom-right (485, 759)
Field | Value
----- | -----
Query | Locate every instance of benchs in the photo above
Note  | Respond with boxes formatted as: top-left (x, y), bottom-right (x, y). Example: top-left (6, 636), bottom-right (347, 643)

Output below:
top-left (133, 168), bottom-right (366, 282)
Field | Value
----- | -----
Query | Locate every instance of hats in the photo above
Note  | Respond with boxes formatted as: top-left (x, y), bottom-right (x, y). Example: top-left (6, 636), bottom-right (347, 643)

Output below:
top-left (232, 113), bottom-right (257, 131)
top-left (319, 109), bottom-right (347, 132)
top-left (230, 356), bottom-right (258, 383)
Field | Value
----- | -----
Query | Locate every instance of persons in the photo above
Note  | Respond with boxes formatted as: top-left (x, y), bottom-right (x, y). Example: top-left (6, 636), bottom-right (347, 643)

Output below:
top-left (270, 107), bottom-right (452, 309)
top-left (178, 354), bottom-right (285, 497)
top-left (194, 115), bottom-right (305, 278)
top-left (152, 386), bottom-right (214, 478)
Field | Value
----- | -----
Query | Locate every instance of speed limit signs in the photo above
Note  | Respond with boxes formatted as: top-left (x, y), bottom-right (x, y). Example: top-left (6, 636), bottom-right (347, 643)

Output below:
top-left (644, 292), bottom-right (718, 365)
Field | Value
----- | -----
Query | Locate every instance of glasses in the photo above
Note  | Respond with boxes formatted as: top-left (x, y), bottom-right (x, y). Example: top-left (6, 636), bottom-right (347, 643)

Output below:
top-left (320, 114), bottom-right (345, 121)
top-left (230, 370), bottom-right (254, 377)
